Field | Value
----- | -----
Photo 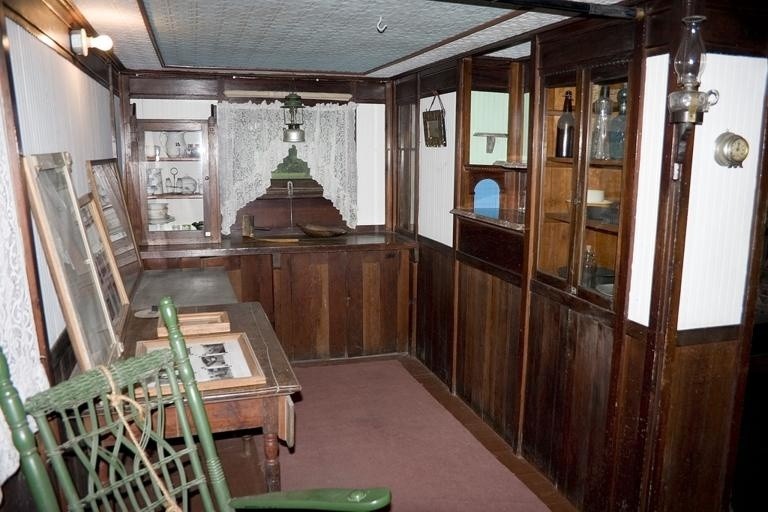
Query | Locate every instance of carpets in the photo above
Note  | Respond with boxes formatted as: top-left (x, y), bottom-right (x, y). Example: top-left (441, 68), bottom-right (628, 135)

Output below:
top-left (278, 359), bottom-right (552, 512)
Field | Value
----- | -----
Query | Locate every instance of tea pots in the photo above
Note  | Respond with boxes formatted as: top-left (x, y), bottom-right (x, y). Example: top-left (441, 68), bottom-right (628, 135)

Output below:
top-left (163, 132), bottom-right (186, 158)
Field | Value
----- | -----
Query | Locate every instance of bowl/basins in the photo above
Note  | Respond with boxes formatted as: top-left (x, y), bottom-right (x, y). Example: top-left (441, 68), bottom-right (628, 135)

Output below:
top-left (566, 200), bottom-right (612, 220)
top-left (596, 284), bottom-right (613, 297)
top-left (147, 203), bottom-right (168, 220)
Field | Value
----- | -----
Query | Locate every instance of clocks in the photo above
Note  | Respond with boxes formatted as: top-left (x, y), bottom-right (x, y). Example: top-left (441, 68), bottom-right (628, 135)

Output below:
top-left (714, 132), bottom-right (749, 168)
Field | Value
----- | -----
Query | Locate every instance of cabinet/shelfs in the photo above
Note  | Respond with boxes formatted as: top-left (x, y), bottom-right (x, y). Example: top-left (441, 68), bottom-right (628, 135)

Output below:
top-left (414, 236), bottom-right (523, 455)
top-left (130, 103), bottom-right (222, 245)
top-left (528, 9), bottom-right (647, 316)
top-left (139, 255), bottom-right (274, 333)
top-left (519, 279), bottom-right (750, 509)
top-left (450, 35), bottom-right (533, 277)
top-left (273, 244), bottom-right (410, 367)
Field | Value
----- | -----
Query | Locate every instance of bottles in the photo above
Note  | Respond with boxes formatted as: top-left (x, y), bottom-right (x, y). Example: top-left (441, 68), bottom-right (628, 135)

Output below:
top-left (556, 90), bottom-right (574, 158)
top-left (176, 178), bottom-right (183, 192)
top-left (609, 83), bottom-right (628, 161)
top-left (582, 244), bottom-right (597, 289)
top-left (591, 86), bottom-right (612, 161)
top-left (165, 178), bottom-right (173, 193)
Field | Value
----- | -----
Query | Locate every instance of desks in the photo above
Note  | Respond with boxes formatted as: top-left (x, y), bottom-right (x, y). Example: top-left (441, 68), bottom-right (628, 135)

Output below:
top-left (57, 303), bottom-right (304, 511)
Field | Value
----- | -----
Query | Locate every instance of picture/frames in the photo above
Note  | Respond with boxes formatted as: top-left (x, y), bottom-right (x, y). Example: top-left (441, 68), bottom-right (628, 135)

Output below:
top-left (423, 110), bottom-right (447, 147)
top-left (156, 311), bottom-right (231, 337)
top-left (131, 332), bottom-right (266, 398)
top-left (77, 192), bottom-right (130, 340)
top-left (22, 152), bottom-right (120, 374)
top-left (86, 158), bottom-right (142, 268)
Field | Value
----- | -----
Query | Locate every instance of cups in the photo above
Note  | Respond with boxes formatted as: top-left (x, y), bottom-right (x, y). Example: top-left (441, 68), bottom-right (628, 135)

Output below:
top-left (587, 190), bottom-right (605, 202)
top-left (242, 215), bottom-right (254, 237)
top-left (147, 186), bottom-right (156, 196)
top-left (145, 145), bottom-right (161, 161)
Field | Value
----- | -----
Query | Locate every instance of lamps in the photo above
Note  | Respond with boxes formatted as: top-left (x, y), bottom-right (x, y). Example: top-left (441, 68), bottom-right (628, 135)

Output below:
top-left (69, 28), bottom-right (113, 57)
top-left (280, 72), bottom-right (305, 143)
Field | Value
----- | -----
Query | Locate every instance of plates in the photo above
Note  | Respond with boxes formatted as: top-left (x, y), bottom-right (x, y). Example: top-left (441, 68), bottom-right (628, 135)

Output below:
top-left (181, 176), bottom-right (197, 195)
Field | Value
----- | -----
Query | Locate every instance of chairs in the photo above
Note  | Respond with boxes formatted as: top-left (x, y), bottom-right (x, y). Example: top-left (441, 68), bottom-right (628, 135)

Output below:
top-left (0, 296), bottom-right (391, 512)
